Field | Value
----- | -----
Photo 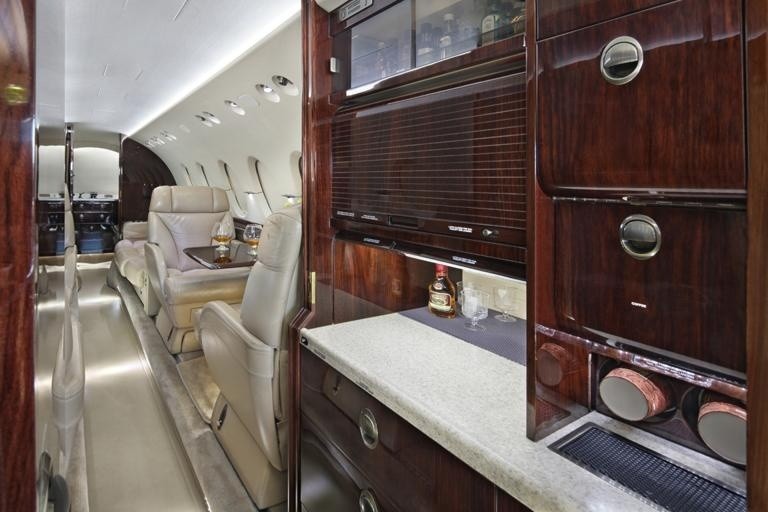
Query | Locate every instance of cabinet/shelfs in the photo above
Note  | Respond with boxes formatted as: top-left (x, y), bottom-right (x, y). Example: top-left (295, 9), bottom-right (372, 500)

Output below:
top-left (527, 0), bottom-right (768, 512)
top-left (324, 0), bottom-right (528, 282)
top-left (299, 346), bottom-right (532, 512)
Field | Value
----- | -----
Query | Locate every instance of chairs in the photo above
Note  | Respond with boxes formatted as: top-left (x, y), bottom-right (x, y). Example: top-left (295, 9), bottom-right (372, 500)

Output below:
top-left (113, 186), bottom-right (259, 354)
top-left (199, 202), bottom-right (302, 508)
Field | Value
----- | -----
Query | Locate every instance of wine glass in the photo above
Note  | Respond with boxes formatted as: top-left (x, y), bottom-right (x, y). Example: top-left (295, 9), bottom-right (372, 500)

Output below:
top-left (212, 250), bottom-right (233, 264)
top-left (211, 222), bottom-right (234, 252)
top-left (456, 280), bottom-right (479, 318)
top-left (492, 285), bottom-right (520, 324)
top-left (241, 223), bottom-right (263, 256)
top-left (459, 288), bottom-right (490, 331)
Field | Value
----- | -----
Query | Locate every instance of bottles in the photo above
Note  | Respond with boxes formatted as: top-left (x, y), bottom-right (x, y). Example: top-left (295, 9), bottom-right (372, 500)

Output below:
top-left (370, 0), bottom-right (527, 87)
top-left (427, 263), bottom-right (456, 319)
